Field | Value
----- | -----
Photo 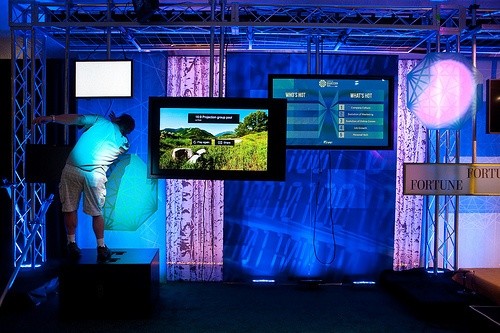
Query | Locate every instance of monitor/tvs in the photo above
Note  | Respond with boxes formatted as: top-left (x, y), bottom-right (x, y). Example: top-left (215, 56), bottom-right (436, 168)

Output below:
top-left (486, 79), bottom-right (500, 134)
top-left (268, 74), bottom-right (395, 150)
top-left (72, 59), bottom-right (134, 98)
top-left (147, 96), bottom-right (287, 181)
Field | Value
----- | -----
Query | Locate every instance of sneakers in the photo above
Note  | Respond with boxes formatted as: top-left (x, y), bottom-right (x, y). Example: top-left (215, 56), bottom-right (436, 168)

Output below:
top-left (97, 245), bottom-right (111, 260)
top-left (63, 242), bottom-right (82, 258)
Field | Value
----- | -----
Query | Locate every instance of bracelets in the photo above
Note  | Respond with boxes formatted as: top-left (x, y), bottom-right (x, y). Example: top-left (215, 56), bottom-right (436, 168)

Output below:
top-left (53, 115), bottom-right (55, 122)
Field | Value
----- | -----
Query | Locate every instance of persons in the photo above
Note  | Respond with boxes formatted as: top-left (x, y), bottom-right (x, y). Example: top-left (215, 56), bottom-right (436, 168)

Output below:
top-left (35, 111), bottom-right (135, 258)
